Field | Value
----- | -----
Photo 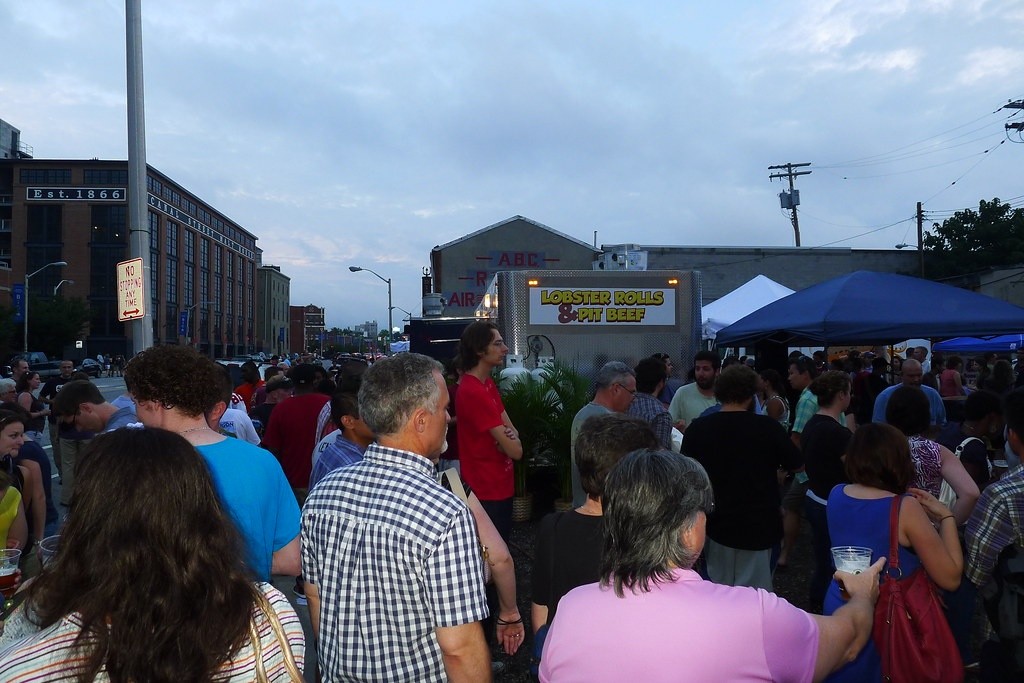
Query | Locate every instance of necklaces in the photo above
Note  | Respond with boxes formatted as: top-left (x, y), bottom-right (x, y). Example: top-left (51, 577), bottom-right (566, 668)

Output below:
top-left (177, 427), bottom-right (210, 436)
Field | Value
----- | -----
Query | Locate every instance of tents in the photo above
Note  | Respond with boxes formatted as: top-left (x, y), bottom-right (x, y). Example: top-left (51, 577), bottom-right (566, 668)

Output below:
top-left (716, 270), bottom-right (1024, 386)
top-left (702, 274), bottom-right (824, 357)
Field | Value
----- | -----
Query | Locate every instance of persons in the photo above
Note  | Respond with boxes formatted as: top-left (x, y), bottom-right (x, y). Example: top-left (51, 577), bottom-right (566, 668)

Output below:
top-left (0, 320), bottom-right (1024, 683)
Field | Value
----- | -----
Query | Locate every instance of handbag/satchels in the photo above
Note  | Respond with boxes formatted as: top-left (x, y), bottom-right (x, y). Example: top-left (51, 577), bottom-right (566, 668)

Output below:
top-left (876, 570), bottom-right (965, 683)
top-left (939, 436), bottom-right (995, 526)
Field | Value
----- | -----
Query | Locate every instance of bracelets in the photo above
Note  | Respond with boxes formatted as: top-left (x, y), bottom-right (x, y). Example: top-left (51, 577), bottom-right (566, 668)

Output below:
top-left (34, 539), bottom-right (41, 546)
top-left (940, 515), bottom-right (955, 522)
top-left (496, 617), bottom-right (523, 625)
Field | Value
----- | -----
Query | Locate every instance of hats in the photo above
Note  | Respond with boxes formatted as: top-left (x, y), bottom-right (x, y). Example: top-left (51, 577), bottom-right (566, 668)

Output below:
top-left (264, 376), bottom-right (294, 393)
top-left (235, 360), bottom-right (262, 377)
top-left (870, 355), bottom-right (892, 368)
top-left (291, 363), bottom-right (316, 385)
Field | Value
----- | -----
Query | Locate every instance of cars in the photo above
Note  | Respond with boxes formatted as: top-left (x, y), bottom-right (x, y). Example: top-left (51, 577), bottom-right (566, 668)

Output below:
top-left (231, 352), bottom-right (299, 362)
top-left (332, 352), bottom-right (383, 362)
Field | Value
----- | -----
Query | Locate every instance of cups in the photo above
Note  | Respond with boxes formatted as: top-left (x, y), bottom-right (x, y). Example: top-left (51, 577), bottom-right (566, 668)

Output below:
top-left (0, 548), bottom-right (22, 591)
top-left (831, 546), bottom-right (873, 601)
top-left (39, 535), bottom-right (63, 570)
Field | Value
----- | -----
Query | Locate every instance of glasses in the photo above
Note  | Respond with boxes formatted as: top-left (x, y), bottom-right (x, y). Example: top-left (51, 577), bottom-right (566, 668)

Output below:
top-left (8, 389), bottom-right (17, 394)
top-left (612, 381), bottom-right (641, 401)
top-left (798, 355), bottom-right (807, 366)
top-left (68, 409), bottom-right (79, 429)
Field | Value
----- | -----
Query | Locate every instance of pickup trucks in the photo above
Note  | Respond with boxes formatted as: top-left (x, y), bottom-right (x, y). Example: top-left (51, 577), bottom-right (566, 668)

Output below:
top-left (1, 353), bottom-right (103, 383)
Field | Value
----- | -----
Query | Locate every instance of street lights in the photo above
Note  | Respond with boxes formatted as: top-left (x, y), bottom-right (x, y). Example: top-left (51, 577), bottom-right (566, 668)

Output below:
top-left (895, 243), bottom-right (925, 279)
top-left (185, 301), bottom-right (215, 345)
top-left (349, 266), bottom-right (392, 343)
top-left (24, 262), bottom-right (67, 352)
top-left (388, 306), bottom-right (411, 324)
top-left (53, 280), bottom-right (74, 301)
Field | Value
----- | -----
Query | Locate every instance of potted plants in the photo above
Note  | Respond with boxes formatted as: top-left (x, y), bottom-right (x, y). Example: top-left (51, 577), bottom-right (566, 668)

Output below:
top-left (498, 357), bottom-right (593, 522)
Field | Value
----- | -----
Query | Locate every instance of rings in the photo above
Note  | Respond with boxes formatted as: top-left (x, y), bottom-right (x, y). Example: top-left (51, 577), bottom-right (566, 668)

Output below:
top-left (515, 634), bottom-right (519, 637)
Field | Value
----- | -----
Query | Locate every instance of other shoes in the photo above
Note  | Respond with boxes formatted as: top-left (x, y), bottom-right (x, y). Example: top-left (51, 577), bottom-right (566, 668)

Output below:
top-left (291, 584), bottom-right (307, 599)
top-left (491, 660), bottom-right (507, 676)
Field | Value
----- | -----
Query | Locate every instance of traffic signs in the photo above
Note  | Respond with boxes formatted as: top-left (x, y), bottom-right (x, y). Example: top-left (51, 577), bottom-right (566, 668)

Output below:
top-left (116, 257), bottom-right (144, 321)
